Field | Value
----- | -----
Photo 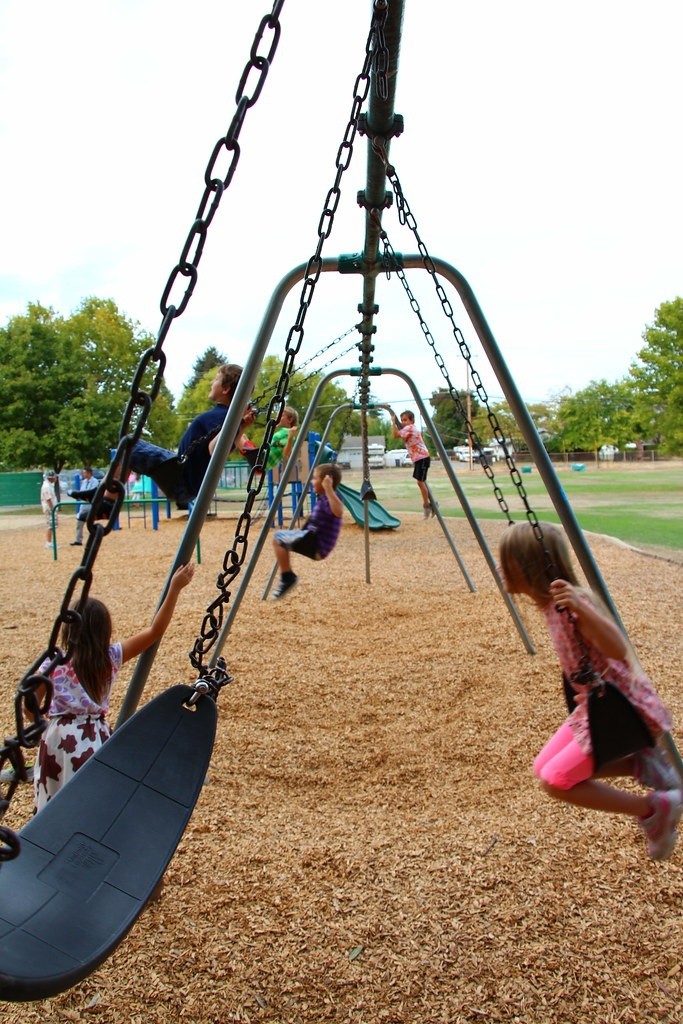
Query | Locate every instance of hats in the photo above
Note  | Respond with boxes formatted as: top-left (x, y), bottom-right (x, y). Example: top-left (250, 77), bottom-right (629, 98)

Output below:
top-left (43, 469), bottom-right (59, 479)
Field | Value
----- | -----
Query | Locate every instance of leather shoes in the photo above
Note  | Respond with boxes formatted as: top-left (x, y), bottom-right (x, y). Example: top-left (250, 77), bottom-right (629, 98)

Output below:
top-left (70, 541), bottom-right (82, 546)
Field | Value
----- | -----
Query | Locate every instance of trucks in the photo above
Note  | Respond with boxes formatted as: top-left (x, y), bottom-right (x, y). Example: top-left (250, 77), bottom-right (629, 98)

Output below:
top-left (367, 443), bottom-right (386, 468)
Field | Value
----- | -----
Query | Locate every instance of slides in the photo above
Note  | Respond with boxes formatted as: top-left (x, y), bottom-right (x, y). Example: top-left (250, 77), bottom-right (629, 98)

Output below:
top-left (333, 479), bottom-right (400, 532)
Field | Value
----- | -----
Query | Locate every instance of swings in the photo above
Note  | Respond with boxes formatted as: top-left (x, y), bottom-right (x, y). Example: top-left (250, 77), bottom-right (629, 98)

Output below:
top-left (1, 2), bottom-right (663, 1003)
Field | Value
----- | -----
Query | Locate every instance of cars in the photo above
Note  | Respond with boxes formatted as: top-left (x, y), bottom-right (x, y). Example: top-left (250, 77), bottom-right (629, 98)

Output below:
top-left (402, 453), bottom-right (413, 467)
top-left (454, 446), bottom-right (498, 463)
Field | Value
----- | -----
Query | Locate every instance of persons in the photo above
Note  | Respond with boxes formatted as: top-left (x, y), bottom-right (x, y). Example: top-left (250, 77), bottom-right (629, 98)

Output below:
top-left (490, 519), bottom-right (683, 863)
top-left (386, 408), bottom-right (442, 519)
top-left (67, 362), bottom-right (258, 528)
top-left (40, 469), bottom-right (63, 552)
top-left (25, 559), bottom-right (201, 811)
top-left (68, 467), bottom-right (102, 547)
top-left (268, 462), bottom-right (345, 606)
top-left (233, 404), bottom-right (300, 476)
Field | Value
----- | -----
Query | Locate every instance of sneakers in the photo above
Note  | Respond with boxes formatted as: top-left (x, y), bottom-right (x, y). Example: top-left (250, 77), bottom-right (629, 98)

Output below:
top-left (633, 740), bottom-right (683, 860)
top-left (271, 574), bottom-right (297, 600)
top-left (46, 541), bottom-right (60, 549)
top-left (423, 502), bottom-right (439, 520)
top-left (67, 486), bottom-right (113, 521)
